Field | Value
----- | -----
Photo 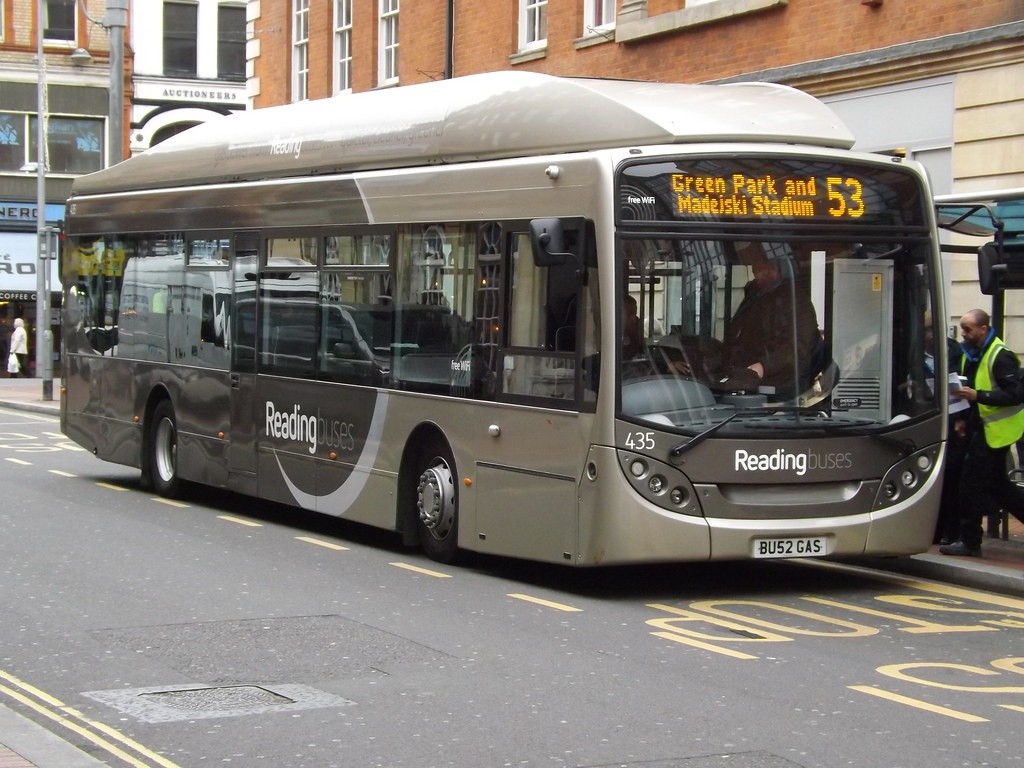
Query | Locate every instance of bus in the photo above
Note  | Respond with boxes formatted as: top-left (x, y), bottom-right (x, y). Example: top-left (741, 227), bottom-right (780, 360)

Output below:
top-left (60, 70), bottom-right (1024, 570)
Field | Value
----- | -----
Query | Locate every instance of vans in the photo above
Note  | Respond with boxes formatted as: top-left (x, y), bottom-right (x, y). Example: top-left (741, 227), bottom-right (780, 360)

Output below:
top-left (232, 296), bottom-right (497, 402)
top-left (117, 255), bottom-right (320, 371)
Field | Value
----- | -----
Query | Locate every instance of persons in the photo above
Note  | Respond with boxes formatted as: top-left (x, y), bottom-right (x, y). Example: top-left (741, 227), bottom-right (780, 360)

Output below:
top-left (7, 318), bottom-right (34, 378)
top-left (731, 247), bottom-right (818, 402)
top-left (923, 310), bottom-right (964, 544)
top-left (621, 295), bottom-right (689, 382)
top-left (940, 309), bottom-right (1024, 556)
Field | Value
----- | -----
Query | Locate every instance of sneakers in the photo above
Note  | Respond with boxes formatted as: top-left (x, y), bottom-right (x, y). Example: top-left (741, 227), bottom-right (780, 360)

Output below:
top-left (938, 537), bottom-right (985, 558)
